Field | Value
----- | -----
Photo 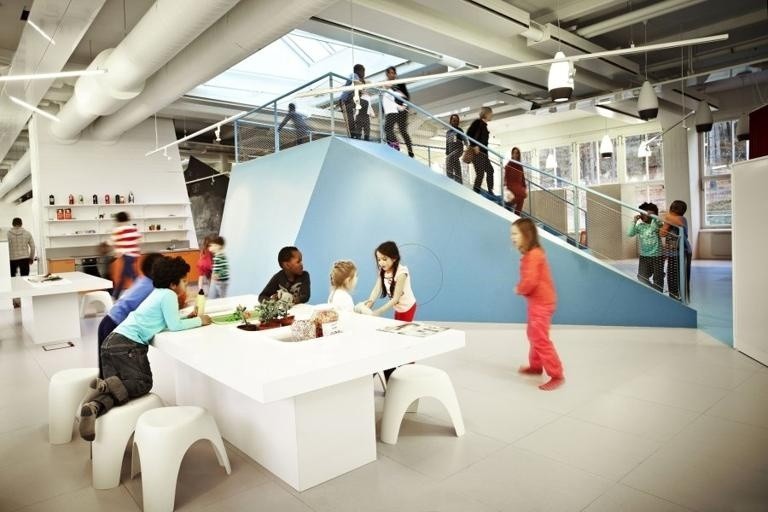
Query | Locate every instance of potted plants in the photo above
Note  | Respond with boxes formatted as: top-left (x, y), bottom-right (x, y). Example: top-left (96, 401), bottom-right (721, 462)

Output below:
top-left (232, 295), bottom-right (295, 333)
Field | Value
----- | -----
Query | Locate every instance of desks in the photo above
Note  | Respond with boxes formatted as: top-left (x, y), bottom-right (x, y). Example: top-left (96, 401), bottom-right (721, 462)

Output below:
top-left (4, 270), bottom-right (114, 350)
top-left (148, 296), bottom-right (468, 491)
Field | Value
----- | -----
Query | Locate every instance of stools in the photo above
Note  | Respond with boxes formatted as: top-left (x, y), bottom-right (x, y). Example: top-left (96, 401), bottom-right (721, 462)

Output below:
top-left (130, 404), bottom-right (230, 508)
top-left (381, 365), bottom-right (466, 446)
top-left (85, 393), bottom-right (163, 488)
top-left (81, 291), bottom-right (111, 317)
top-left (45, 367), bottom-right (103, 446)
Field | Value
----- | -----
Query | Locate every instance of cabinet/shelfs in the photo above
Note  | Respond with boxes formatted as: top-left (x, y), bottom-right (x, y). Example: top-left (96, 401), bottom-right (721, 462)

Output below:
top-left (42, 201), bottom-right (202, 285)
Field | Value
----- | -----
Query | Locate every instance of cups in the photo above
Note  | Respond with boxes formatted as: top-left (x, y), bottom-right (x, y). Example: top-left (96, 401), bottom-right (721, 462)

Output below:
top-left (162, 224), bottom-right (167, 231)
top-left (177, 223), bottom-right (183, 229)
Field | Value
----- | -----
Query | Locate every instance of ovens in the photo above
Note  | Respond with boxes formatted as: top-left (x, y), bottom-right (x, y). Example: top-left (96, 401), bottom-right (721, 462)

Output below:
top-left (76, 264), bottom-right (108, 278)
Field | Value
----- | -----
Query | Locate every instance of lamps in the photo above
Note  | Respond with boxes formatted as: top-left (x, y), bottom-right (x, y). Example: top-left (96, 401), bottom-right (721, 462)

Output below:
top-left (546, 0), bottom-right (575, 104)
top-left (599, 119), bottom-right (614, 158)
top-left (733, 113), bottom-right (749, 142)
top-left (637, 19), bottom-right (659, 121)
top-left (692, 49), bottom-right (714, 132)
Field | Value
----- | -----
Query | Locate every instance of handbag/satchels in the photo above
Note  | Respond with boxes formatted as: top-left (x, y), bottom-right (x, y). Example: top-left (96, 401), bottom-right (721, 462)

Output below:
top-left (503, 186), bottom-right (515, 202)
top-left (463, 146), bottom-right (475, 164)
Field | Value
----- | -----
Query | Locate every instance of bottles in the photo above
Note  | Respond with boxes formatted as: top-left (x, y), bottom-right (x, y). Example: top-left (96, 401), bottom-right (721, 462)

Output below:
top-left (64, 208), bottom-right (71, 219)
top-left (104, 193), bottom-right (109, 204)
top-left (114, 191), bottom-right (133, 205)
top-left (48, 194), bottom-right (54, 205)
top-left (78, 194), bottom-right (83, 204)
top-left (92, 193), bottom-right (98, 204)
top-left (193, 288), bottom-right (205, 317)
top-left (149, 224), bottom-right (161, 231)
top-left (56, 208), bottom-right (63, 220)
top-left (68, 194), bottom-right (74, 205)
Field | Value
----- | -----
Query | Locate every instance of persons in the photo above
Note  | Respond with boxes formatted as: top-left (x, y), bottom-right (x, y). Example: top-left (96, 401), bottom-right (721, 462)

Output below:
top-left (365, 241), bottom-right (416, 323)
top-left (111, 211), bottom-right (142, 298)
top-left (255, 246), bottom-right (310, 305)
top-left (657, 200), bottom-right (692, 305)
top-left (207, 236), bottom-right (229, 299)
top-left (325, 259), bottom-right (358, 313)
top-left (97, 240), bottom-right (117, 282)
top-left (276, 63), bottom-right (528, 216)
top-left (78, 255), bottom-right (212, 442)
top-left (91, 252), bottom-right (165, 388)
top-left (625, 202), bottom-right (665, 293)
top-left (6, 217), bottom-right (36, 277)
top-left (197, 232), bottom-right (211, 295)
top-left (509, 217), bottom-right (565, 392)
